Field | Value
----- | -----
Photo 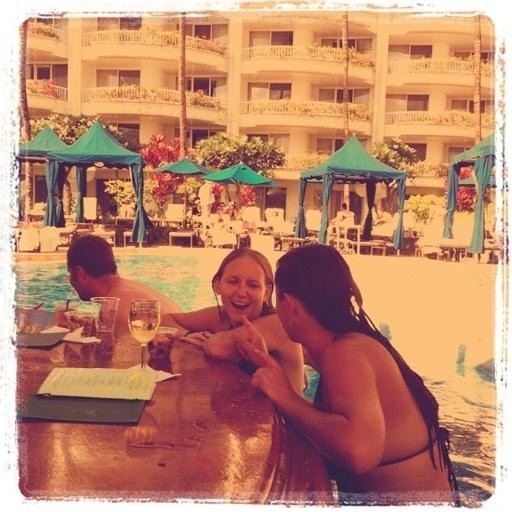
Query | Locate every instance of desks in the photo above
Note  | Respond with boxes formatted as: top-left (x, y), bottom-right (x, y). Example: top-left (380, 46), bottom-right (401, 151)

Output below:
top-left (15, 307), bottom-right (340, 504)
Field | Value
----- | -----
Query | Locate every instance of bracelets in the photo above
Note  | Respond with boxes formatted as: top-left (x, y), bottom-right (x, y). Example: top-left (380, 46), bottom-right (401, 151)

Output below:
top-left (183, 331), bottom-right (192, 336)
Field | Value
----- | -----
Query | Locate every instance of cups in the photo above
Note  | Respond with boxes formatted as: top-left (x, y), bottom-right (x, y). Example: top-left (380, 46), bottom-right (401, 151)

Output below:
top-left (53, 297), bottom-right (121, 339)
top-left (146, 326), bottom-right (178, 370)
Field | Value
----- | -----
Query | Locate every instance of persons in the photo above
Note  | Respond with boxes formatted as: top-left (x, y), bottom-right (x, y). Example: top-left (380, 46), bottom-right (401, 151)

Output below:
top-left (210, 195), bottom-right (221, 212)
top-left (67, 234), bottom-right (185, 315)
top-left (238, 243), bottom-right (459, 506)
top-left (192, 196), bottom-right (201, 214)
top-left (160, 247), bottom-right (310, 394)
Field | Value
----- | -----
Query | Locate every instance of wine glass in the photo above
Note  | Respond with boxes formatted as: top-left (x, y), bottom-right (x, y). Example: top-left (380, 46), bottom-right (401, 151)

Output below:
top-left (127, 299), bottom-right (160, 369)
